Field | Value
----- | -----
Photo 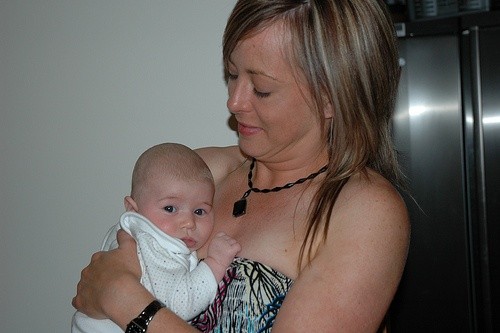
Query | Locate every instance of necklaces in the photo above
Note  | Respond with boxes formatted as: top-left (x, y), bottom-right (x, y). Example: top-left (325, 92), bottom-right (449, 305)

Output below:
top-left (232, 157), bottom-right (329, 217)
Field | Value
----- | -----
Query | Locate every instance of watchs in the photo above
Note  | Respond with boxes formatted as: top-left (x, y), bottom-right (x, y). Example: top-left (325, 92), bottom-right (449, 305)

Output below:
top-left (125, 300), bottom-right (166, 333)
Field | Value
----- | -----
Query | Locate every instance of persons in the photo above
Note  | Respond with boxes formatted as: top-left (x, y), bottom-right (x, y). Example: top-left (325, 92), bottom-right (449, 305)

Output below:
top-left (72, 141), bottom-right (242, 333)
top-left (72, 0), bottom-right (413, 333)
